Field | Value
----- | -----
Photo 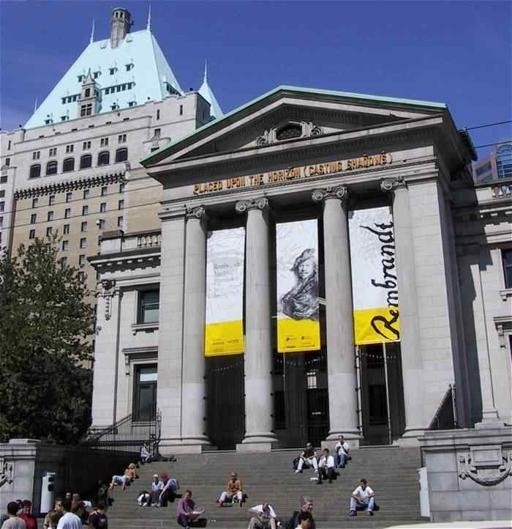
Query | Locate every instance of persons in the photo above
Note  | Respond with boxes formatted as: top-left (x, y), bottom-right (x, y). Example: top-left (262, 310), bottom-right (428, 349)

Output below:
top-left (281, 249), bottom-right (321, 321)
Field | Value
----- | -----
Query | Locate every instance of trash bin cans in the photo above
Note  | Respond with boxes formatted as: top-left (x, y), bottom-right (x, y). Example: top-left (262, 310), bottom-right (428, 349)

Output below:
top-left (417, 467), bottom-right (431, 518)
top-left (40, 472), bottom-right (56, 514)
